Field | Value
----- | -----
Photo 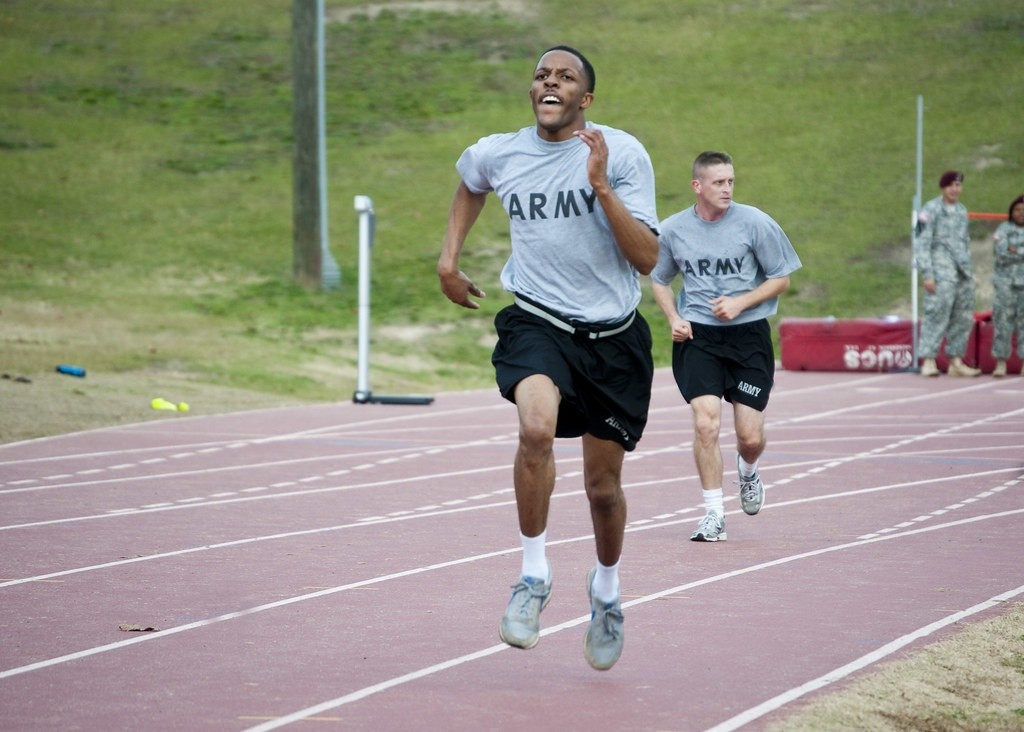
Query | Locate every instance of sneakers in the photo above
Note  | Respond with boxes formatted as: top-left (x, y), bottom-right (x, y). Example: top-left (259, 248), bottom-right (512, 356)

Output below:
top-left (498, 559), bottom-right (552, 650)
top-left (690, 510), bottom-right (728, 542)
top-left (733, 453), bottom-right (765, 515)
top-left (583, 566), bottom-right (624, 670)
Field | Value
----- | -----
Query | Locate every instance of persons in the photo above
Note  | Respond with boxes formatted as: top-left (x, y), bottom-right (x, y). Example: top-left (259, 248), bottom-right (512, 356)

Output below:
top-left (647, 148), bottom-right (803, 544)
top-left (915, 171), bottom-right (982, 377)
top-left (437, 44), bottom-right (660, 672)
top-left (991, 194), bottom-right (1024, 376)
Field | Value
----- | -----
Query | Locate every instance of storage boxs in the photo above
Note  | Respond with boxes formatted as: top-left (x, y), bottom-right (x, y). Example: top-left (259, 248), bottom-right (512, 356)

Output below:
top-left (780, 309), bottom-right (1024, 375)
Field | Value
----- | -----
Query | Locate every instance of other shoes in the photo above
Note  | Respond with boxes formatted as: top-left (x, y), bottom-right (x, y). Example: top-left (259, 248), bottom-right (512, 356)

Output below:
top-left (993, 362), bottom-right (1007, 376)
top-left (948, 362), bottom-right (980, 377)
top-left (921, 358), bottom-right (939, 376)
